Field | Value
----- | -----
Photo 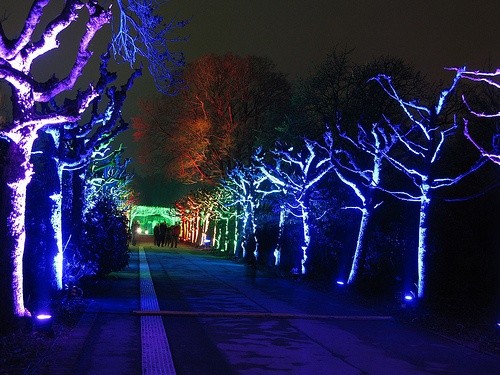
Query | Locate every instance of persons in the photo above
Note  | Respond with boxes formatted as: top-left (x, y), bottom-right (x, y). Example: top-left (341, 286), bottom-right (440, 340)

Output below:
top-left (171, 222), bottom-right (181, 248)
top-left (164, 224), bottom-right (173, 247)
top-left (132, 220), bottom-right (138, 246)
top-left (158, 221), bottom-right (167, 247)
top-left (154, 223), bottom-right (159, 247)
